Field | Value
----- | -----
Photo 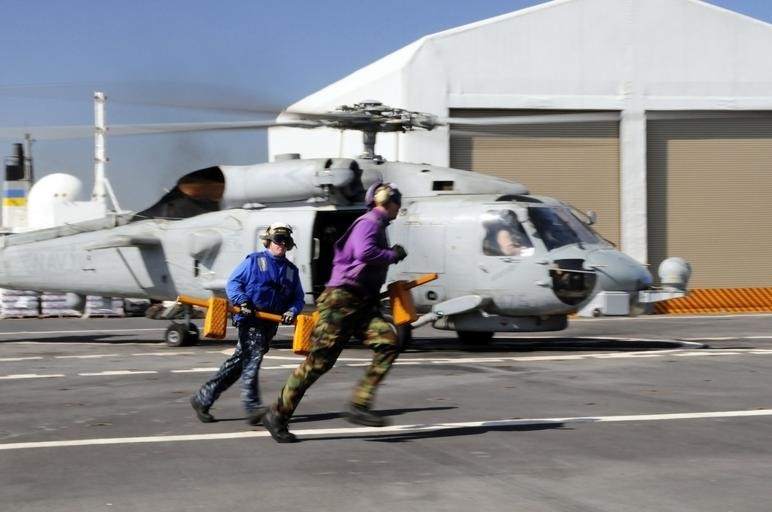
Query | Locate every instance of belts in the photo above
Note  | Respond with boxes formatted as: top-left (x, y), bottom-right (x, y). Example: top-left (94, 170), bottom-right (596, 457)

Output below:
top-left (338, 284), bottom-right (378, 304)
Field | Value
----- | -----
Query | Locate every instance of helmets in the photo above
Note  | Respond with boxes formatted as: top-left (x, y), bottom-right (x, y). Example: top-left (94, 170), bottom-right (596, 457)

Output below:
top-left (365, 181), bottom-right (401, 209)
top-left (265, 221), bottom-right (294, 233)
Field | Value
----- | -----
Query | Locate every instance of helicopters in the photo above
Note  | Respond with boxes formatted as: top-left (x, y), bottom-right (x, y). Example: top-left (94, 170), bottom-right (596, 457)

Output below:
top-left (0, 83), bottom-right (652, 352)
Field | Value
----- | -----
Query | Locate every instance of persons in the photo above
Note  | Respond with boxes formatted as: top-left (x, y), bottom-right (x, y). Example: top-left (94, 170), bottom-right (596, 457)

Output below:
top-left (258, 181), bottom-right (408, 443)
top-left (188, 220), bottom-right (306, 427)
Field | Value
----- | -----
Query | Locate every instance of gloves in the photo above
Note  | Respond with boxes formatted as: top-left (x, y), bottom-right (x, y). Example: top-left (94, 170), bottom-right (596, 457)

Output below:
top-left (240, 302), bottom-right (257, 317)
top-left (392, 244), bottom-right (407, 263)
top-left (281, 310), bottom-right (295, 325)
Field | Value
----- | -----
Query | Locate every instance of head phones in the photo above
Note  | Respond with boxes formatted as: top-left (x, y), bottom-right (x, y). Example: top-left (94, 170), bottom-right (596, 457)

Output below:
top-left (263, 226), bottom-right (293, 250)
top-left (375, 190), bottom-right (389, 204)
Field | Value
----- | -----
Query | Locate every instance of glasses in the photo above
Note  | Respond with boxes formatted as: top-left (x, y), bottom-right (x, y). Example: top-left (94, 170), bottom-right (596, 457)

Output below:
top-left (270, 234), bottom-right (293, 251)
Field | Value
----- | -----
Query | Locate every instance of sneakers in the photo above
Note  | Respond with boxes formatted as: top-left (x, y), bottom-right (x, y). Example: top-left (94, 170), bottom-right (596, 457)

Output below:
top-left (262, 411), bottom-right (297, 443)
top-left (189, 394), bottom-right (214, 423)
top-left (344, 403), bottom-right (384, 426)
top-left (247, 404), bottom-right (270, 425)
